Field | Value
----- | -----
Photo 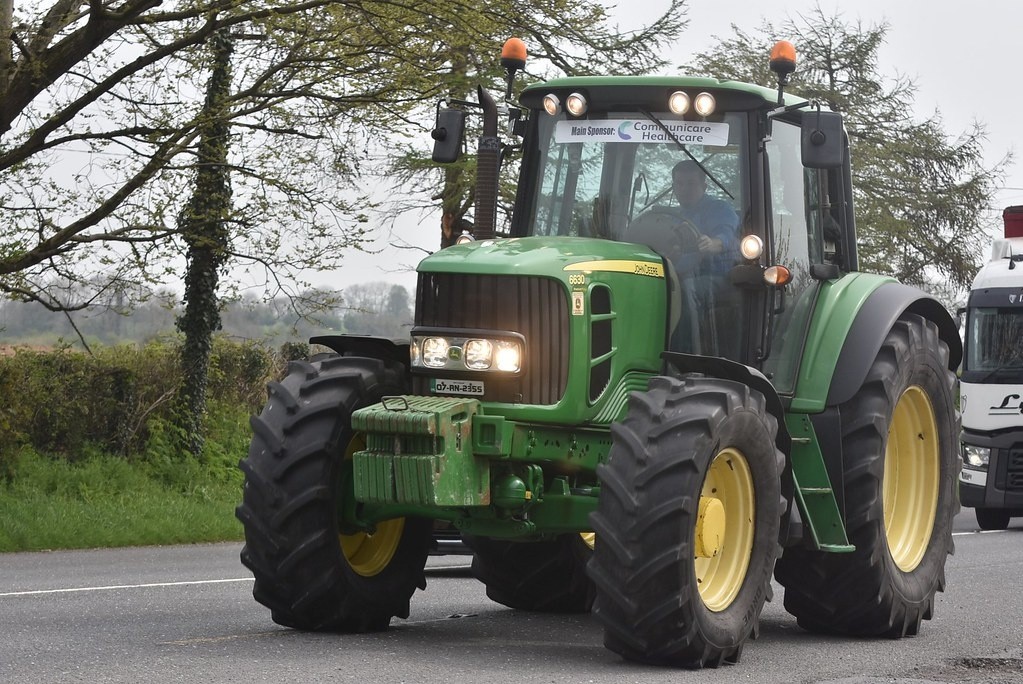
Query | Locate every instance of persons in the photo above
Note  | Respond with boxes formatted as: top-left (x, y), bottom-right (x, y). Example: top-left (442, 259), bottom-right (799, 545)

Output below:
top-left (670, 161), bottom-right (739, 275)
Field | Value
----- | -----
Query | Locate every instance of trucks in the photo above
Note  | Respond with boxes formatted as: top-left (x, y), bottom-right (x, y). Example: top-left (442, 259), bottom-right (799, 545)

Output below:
top-left (944, 200), bottom-right (1022, 530)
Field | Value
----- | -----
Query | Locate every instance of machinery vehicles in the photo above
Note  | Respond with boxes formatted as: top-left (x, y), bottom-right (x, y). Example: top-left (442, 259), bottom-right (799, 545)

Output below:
top-left (232, 34), bottom-right (966, 670)
